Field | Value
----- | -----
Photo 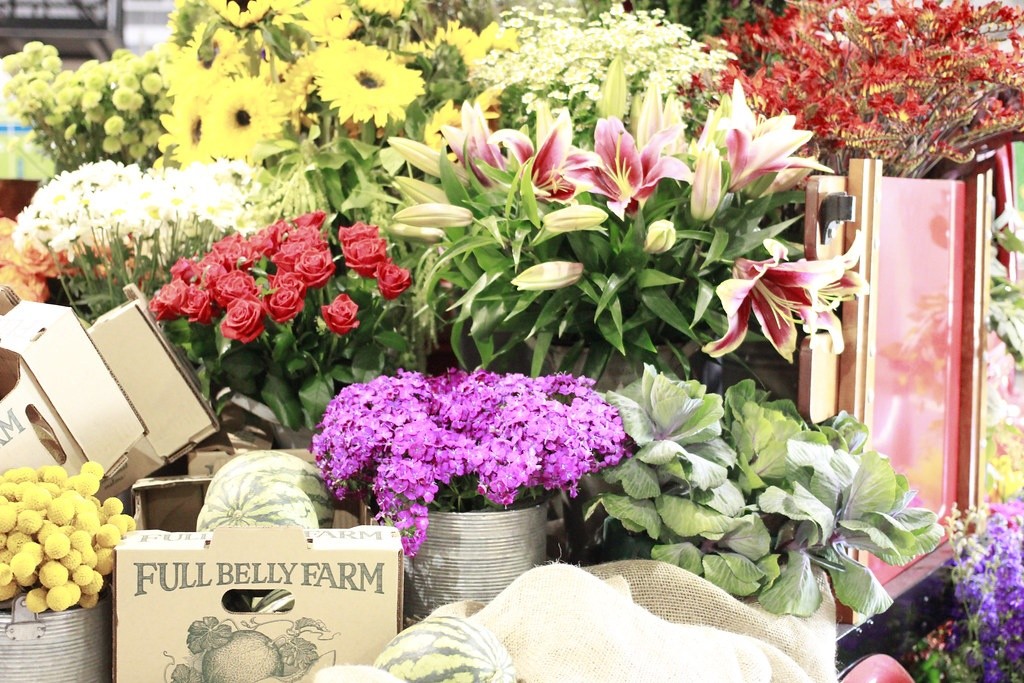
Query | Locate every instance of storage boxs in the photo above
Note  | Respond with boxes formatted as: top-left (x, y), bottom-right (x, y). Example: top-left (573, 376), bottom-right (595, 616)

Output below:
top-left (0, 299), bottom-right (167, 505)
top-left (112, 524), bottom-right (404, 683)
top-left (85, 283), bottom-right (220, 464)
top-left (188, 445), bottom-right (359, 529)
top-left (131, 474), bottom-right (214, 533)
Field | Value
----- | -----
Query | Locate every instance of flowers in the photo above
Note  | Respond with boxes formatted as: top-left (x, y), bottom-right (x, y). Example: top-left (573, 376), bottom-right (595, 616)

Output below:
top-left (0, 0), bottom-right (1024, 683)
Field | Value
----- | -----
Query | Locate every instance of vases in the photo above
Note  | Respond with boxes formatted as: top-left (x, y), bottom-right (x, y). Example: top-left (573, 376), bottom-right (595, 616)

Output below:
top-left (404, 503), bottom-right (548, 620)
top-left (0, 591), bottom-right (112, 683)
